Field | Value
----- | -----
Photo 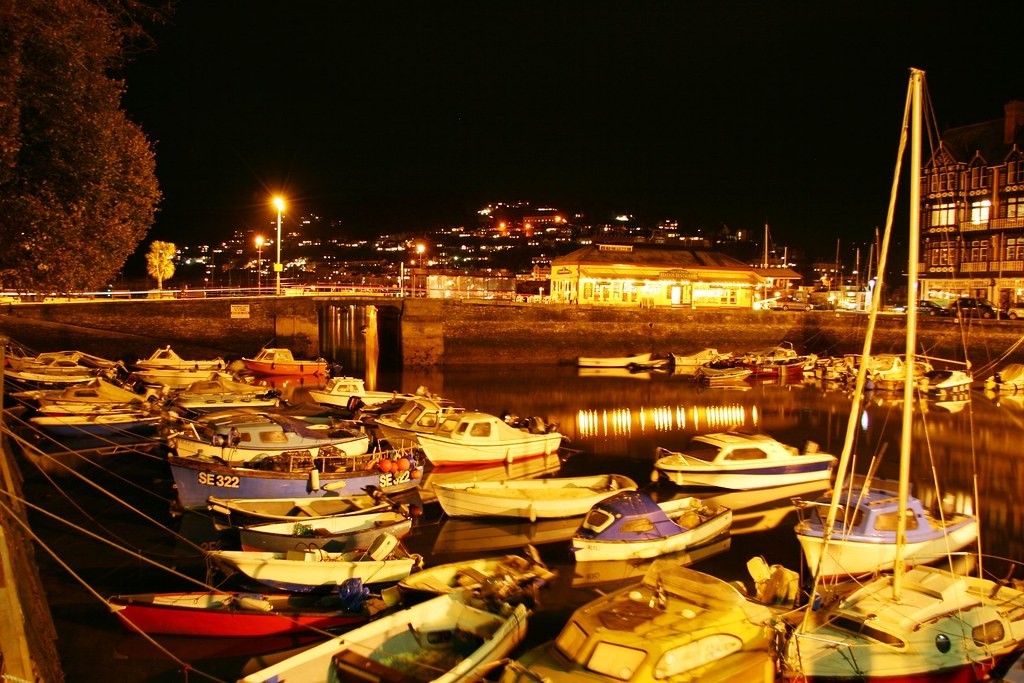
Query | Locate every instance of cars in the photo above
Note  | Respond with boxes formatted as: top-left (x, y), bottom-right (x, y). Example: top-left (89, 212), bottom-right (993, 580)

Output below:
top-left (768, 296), bottom-right (815, 311)
top-left (1008, 302), bottom-right (1024, 321)
top-left (948, 297), bottom-right (1005, 319)
top-left (917, 300), bottom-right (950, 316)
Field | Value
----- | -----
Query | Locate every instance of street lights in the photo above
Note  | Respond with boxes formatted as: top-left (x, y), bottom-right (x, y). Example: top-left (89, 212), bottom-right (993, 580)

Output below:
top-left (274, 196), bottom-right (287, 296)
top-left (256, 235), bottom-right (264, 296)
top-left (418, 243), bottom-right (426, 296)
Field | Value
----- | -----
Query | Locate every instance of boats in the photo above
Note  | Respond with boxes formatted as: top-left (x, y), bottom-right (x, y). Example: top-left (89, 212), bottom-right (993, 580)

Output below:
top-left (2, 63), bottom-right (1024, 683)
